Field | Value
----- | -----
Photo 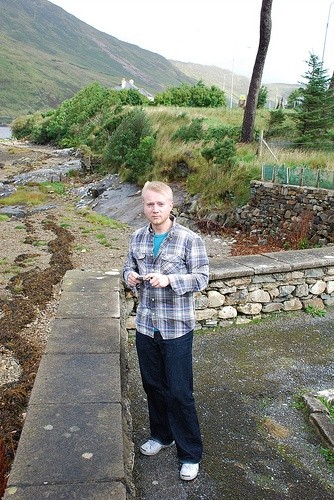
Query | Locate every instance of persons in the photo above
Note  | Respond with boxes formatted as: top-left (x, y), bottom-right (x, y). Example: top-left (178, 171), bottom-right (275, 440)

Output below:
top-left (124, 180), bottom-right (209, 480)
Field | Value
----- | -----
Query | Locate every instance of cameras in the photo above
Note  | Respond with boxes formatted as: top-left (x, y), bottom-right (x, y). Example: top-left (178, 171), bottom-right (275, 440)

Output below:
top-left (137, 276), bottom-right (151, 282)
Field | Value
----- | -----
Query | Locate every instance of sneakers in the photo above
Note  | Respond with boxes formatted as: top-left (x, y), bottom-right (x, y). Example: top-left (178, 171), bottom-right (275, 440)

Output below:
top-left (140, 439), bottom-right (175, 456)
top-left (179, 463), bottom-right (198, 480)
